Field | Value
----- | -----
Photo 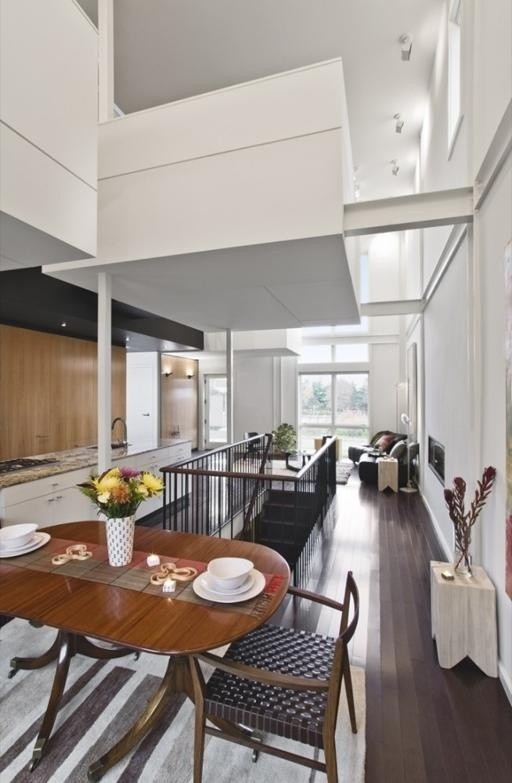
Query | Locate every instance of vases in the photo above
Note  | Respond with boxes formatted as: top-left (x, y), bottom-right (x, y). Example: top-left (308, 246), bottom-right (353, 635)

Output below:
top-left (450, 550), bottom-right (475, 576)
top-left (102, 516), bottom-right (136, 567)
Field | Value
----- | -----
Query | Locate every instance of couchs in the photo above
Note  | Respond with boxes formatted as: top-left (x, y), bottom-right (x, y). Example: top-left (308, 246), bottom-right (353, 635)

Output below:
top-left (348, 431), bottom-right (420, 487)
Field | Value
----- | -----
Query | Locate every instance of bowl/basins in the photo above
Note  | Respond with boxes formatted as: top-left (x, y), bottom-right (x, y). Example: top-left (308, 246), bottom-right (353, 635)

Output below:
top-left (0, 523), bottom-right (38, 546)
top-left (207, 557), bottom-right (254, 588)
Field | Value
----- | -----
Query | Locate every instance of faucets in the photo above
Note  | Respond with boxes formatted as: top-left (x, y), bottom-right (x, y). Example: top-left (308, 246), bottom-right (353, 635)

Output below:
top-left (112, 418), bottom-right (128, 449)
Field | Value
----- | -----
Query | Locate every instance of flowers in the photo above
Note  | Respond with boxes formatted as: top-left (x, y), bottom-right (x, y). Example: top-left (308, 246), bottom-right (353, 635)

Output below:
top-left (75, 466), bottom-right (166, 519)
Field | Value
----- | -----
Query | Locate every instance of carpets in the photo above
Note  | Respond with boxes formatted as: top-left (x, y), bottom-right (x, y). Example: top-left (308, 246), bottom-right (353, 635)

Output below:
top-left (227, 457), bottom-right (354, 485)
top-left (1, 618), bottom-right (365, 783)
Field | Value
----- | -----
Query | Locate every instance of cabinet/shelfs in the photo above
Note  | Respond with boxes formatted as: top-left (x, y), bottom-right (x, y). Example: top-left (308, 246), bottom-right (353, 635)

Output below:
top-left (1, 438), bottom-right (195, 529)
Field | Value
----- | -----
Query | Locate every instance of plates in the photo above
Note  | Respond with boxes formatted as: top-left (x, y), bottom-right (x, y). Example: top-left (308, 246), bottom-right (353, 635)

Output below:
top-left (193, 569), bottom-right (266, 604)
top-left (0, 532), bottom-right (52, 558)
top-left (200, 572), bottom-right (255, 596)
top-left (0, 534), bottom-right (43, 552)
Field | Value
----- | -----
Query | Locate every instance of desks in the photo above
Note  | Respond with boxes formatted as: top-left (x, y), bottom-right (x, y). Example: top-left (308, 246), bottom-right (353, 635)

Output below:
top-left (430, 561), bottom-right (499, 676)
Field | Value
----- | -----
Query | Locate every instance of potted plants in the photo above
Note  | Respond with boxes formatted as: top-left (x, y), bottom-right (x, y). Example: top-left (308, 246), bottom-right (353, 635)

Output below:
top-left (272, 423), bottom-right (298, 460)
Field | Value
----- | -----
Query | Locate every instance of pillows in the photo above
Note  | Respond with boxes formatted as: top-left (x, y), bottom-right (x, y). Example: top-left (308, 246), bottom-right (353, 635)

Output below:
top-left (375, 433), bottom-right (395, 451)
top-left (389, 439), bottom-right (405, 459)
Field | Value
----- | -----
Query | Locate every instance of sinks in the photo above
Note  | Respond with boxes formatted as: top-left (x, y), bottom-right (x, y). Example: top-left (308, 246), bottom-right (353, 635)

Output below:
top-left (88, 443), bottom-right (133, 450)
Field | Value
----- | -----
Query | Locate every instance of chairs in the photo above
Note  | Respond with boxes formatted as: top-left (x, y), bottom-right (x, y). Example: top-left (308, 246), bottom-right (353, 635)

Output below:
top-left (241, 432), bottom-right (262, 461)
top-left (185, 569), bottom-right (361, 783)
